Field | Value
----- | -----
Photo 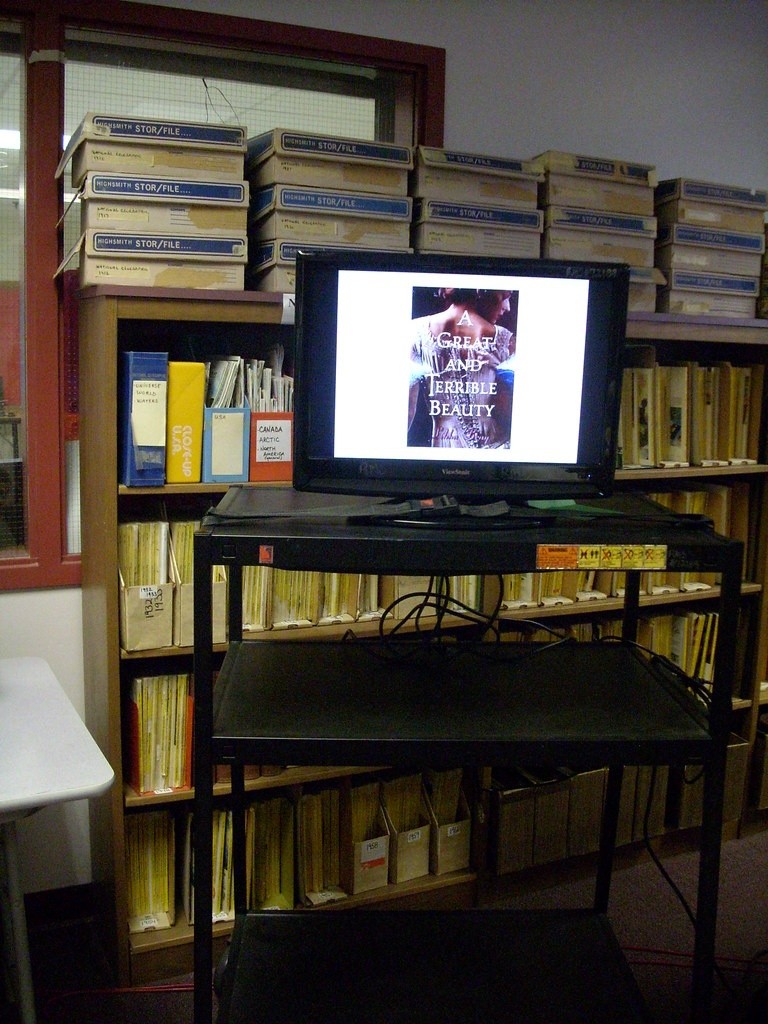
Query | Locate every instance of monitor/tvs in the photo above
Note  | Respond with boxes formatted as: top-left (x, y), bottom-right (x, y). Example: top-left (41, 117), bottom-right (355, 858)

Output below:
top-left (290, 246), bottom-right (631, 530)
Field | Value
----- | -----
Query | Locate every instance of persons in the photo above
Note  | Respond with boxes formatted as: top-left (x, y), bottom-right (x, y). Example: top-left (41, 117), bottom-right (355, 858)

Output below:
top-left (407, 287), bottom-right (516, 448)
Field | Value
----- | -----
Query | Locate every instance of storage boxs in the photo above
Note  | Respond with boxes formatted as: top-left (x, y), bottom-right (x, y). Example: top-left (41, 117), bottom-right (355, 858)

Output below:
top-left (120, 558), bottom-right (227, 651)
top-left (53, 112), bottom-right (768, 316)
top-left (203, 384), bottom-right (296, 483)
top-left (351, 727), bottom-right (749, 893)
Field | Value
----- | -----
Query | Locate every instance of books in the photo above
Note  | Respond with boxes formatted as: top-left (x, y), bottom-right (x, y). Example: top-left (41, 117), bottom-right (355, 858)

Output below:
top-left (206, 355), bottom-right (294, 412)
top-left (619, 360), bottom-right (753, 468)
top-left (122, 490), bottom-right (754, 926)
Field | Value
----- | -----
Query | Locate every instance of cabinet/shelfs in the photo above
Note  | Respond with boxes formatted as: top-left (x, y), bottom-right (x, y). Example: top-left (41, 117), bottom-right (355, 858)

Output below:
top-left (193, 483), bottom-right (747, 1024)
top-left (81, 285), bottom-right (768, 989)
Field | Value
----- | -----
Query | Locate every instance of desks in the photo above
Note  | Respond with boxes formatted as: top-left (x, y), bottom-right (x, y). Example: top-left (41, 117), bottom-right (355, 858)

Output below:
top-left (0, 653), bottom-right (115, 1024)
